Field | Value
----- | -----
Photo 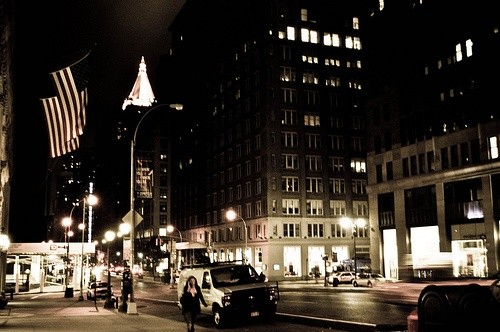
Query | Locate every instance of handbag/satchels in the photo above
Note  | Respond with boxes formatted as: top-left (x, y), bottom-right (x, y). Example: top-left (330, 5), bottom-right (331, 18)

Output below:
top-left (180, 294), bottom-right (184, 304)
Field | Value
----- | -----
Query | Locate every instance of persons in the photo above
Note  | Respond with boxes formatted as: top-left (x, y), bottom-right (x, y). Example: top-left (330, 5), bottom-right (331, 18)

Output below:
top-left (181, 276), bottom-right (208, 332)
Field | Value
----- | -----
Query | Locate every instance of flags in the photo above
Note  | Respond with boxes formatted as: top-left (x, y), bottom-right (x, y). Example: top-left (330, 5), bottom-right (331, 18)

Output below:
top-left (40, 67), bottom-right (88, 159)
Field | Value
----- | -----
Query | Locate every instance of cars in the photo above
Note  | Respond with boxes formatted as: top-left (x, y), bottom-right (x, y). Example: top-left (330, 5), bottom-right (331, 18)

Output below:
top-left (326, 271), bottom-right (358, 284)
top-left (352, 272), bottom-right (386, 288)
top-left (87, 281), bottom-right (114, 301)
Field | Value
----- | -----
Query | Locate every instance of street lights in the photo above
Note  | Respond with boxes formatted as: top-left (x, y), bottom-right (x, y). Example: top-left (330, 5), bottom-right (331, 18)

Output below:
top-left (104, 230), bottom-right (116, 309)
top-left (66, 195), bottom-right (98, 291)
top-left (126, 104), bottom-right (184, 315)
top-left (321, 253), bottom-right (329, 287)
top-left (166, 224), bottom-right (182, 242)
top-left (340, 217), bottom-right (367, 280)
top-left (226, 210), bottom-right (247, 264)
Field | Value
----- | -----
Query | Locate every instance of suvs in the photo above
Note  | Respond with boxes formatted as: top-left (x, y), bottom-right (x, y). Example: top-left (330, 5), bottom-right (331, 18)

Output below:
top-left (177, 262), bottom-right (279, 330)
